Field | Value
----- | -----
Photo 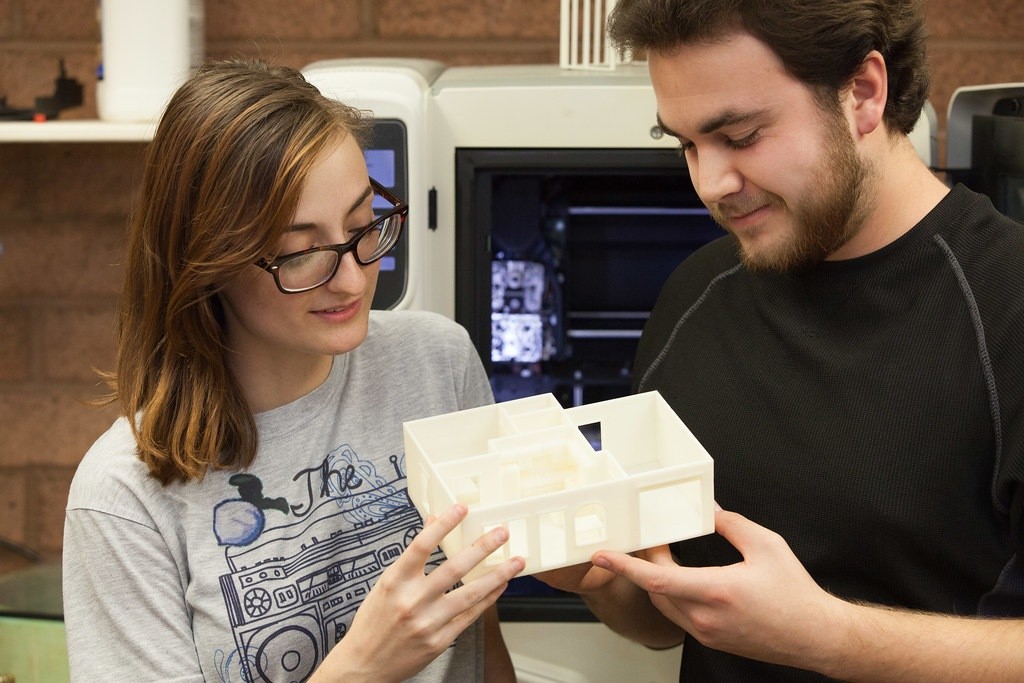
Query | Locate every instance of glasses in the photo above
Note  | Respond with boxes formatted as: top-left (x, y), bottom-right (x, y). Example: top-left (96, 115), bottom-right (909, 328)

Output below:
top-left (223, 175), bottom-right (409, 294)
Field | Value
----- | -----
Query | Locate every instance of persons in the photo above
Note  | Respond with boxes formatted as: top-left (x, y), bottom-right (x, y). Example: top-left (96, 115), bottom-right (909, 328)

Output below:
top-left (63, 62), bottom-right (527, 683)
top-left (530, 1), bottom-right (1024, 683)
